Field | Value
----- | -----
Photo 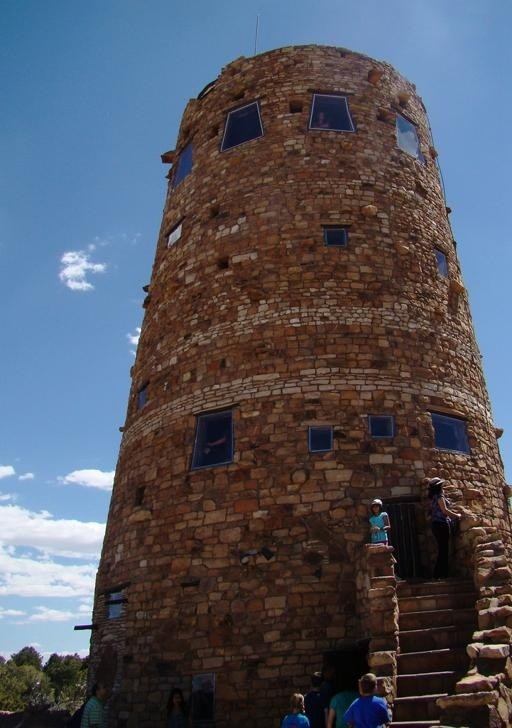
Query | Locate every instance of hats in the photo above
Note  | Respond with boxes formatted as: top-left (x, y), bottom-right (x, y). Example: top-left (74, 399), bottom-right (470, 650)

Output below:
top-left (426, 477), bottom-right (445, 489)
top-left (372, 498), bottom-right (383, 507)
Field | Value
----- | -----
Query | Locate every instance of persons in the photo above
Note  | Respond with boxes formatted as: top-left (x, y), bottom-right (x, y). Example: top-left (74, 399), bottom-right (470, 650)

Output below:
top-left (188, 678), bottom-right (215, 727)
top-left (168, 673), bottom-right (196, 728)
top-left (199, 421), bottom-right (231, 465)
top-left (303, 672), bottom-right (329, 728)
top-left (168, 692), bottom-right (186, 728)
top-left (344, 673), bottom-right (392, 728)
top-left (368, 498), bottom-right (391, 546)
top-left (281, 693), bottom-right (310, 728)
top-left (81, 678), bottom-right (109, 728)
top-left (428, 477), bottom-right (462, 579)
top-left (312, 110), bottom-right (330, 128)
top-left (327, 680), bottom-right (359, 728)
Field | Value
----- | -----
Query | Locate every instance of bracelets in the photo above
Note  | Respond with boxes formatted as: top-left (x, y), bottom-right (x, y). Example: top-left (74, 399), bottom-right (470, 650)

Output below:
top-left (380, 527), bottom-right (383, 530)
